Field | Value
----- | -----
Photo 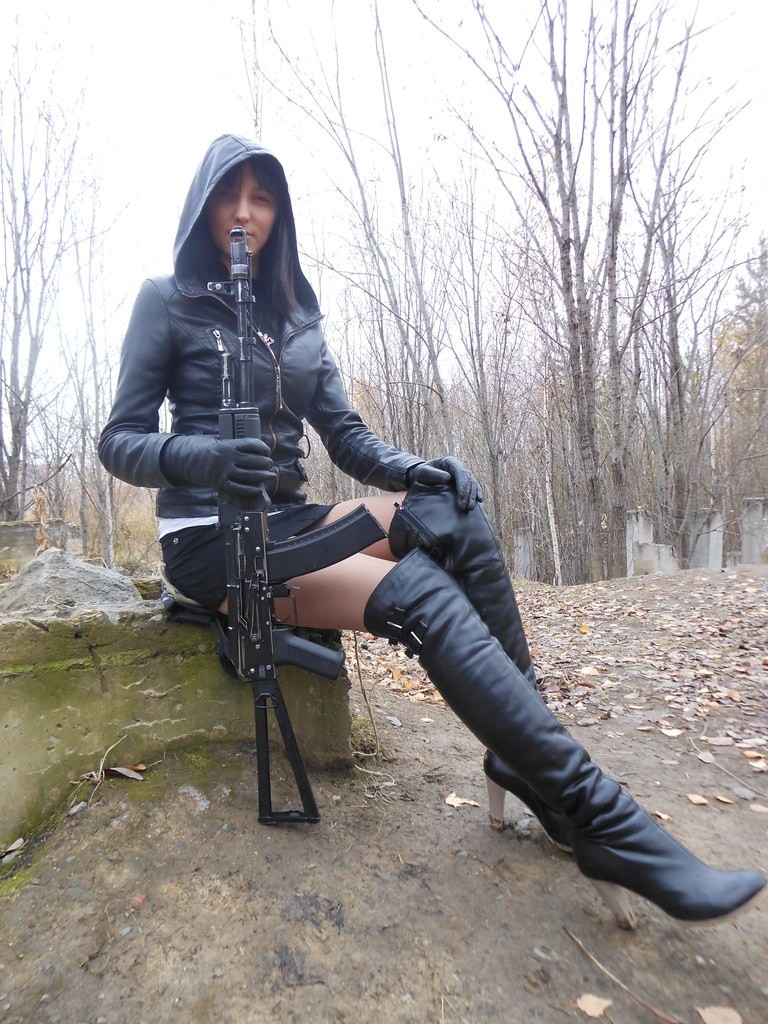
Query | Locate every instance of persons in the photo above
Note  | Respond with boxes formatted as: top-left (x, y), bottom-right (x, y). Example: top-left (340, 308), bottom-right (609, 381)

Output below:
top-left (96, 134), bottom-right (767, 929)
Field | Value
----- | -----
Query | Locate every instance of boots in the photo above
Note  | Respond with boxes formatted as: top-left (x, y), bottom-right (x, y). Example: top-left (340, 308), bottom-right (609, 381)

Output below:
top-left (392, 481), bottom-right (579, 853)
top-left (363, 547), bottom-right (768, 932)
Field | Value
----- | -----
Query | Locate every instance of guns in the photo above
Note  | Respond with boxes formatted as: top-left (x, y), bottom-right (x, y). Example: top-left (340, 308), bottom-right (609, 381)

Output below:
top-left (214, 224), bottom-right (347, 824)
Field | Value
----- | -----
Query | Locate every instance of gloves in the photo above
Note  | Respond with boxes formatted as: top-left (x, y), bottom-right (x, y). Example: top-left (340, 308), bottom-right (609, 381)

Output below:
top-left (165, 437), bottom-right (278, 497)
top-left (406, 456), bottom-right (483, 511)
